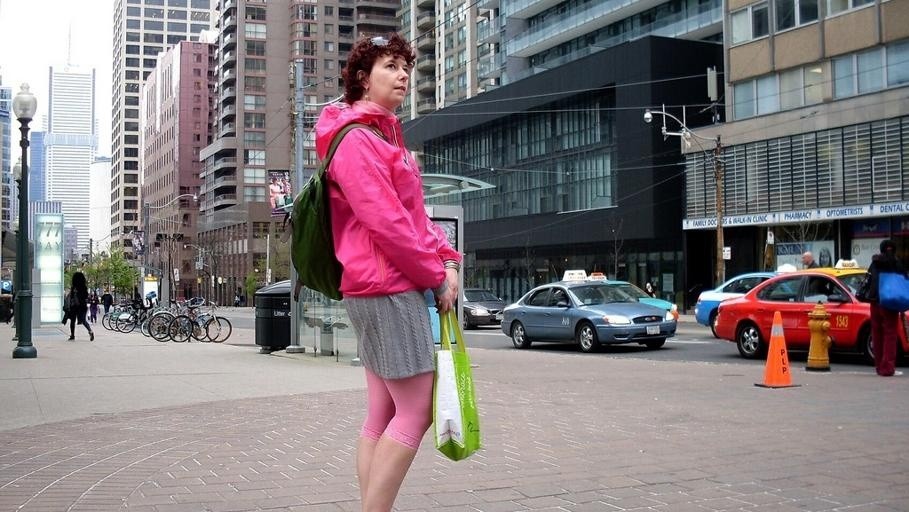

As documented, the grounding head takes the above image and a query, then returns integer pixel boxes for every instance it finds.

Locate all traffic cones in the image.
[755,311,802,388]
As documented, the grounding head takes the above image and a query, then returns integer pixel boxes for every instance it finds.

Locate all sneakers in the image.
[67,319,97,342]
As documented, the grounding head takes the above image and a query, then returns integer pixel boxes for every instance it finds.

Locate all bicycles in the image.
[102,292,232,343]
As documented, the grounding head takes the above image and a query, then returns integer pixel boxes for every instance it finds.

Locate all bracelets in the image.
[433,280,448,295]
[443,260,461,272]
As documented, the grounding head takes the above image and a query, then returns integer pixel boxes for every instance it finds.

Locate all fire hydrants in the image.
[805,301,832,371]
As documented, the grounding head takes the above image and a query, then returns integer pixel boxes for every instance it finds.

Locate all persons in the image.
[868,239,904,376]
[67,272,95,342]
[280,177,292,205]
[100,288,113,319]
[645,282,656,299]
[800,251,819,270]
[269,176,281,212]
[313,30,464,512]
[88,290,99,323]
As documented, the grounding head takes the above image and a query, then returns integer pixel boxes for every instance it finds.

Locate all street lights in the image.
[12,83,37,358]
[144,193,198,264]
[643,109,724,289]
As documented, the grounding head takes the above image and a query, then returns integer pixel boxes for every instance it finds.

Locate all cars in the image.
[463,270,678,352]
[695,259,909,367]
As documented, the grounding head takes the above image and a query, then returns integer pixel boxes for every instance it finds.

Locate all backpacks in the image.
[283,122,388,304]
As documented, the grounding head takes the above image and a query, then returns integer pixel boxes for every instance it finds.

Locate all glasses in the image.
[358,34,392,49]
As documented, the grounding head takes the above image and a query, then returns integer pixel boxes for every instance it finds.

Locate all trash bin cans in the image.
[254,279,292,354]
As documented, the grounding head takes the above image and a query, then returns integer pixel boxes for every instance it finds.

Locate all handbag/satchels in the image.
[428,297,483,462]
